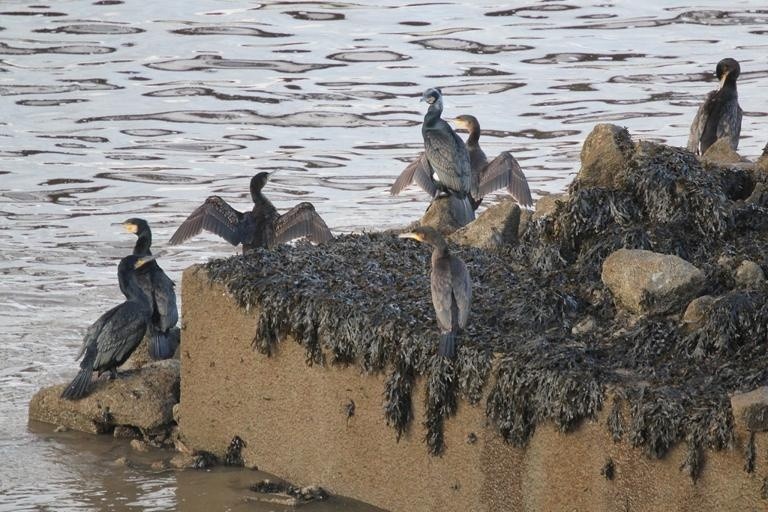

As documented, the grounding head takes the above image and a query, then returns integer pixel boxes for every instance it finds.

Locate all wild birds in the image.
[686,57,744,156]
[60,249,168,401]
[110,217,179,361]
[388,88,534,226]
[167,170,337,257]
[396,224,474,356]
